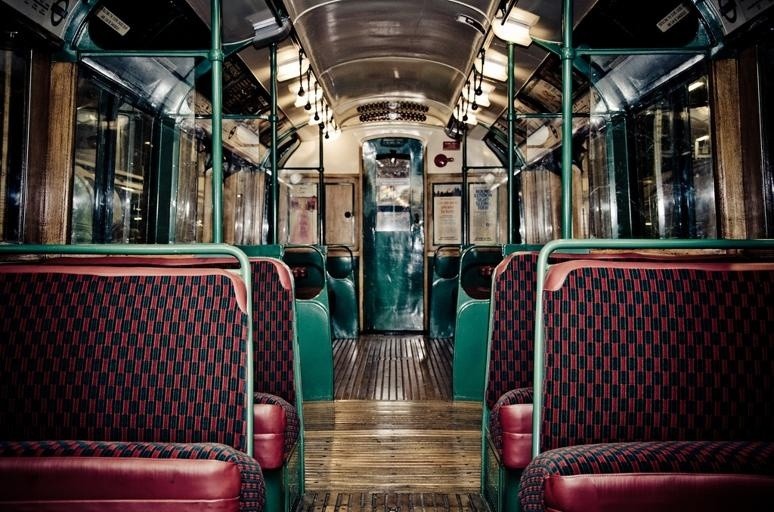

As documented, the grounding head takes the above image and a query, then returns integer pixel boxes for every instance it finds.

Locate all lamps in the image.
[461,84,491,107]
[491,2,538,47]
[468,74,496,94]
[474,48,508,81]
[453,109,478,126]
[277,44,341,142]
[457,96,483,116]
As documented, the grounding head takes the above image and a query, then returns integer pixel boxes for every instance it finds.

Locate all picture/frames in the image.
[432,182,464,246]
[287,182,318,247]
[468,182,499,245]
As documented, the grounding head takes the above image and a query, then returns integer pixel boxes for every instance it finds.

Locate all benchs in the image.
[229,244,336,401]
[429,242,503,338]
[0,265,267,511]
[453,244,543,402]
[480,251,768,510]
[0,241,304,508]
[283,241,359,338]
[518,259,770,510]
